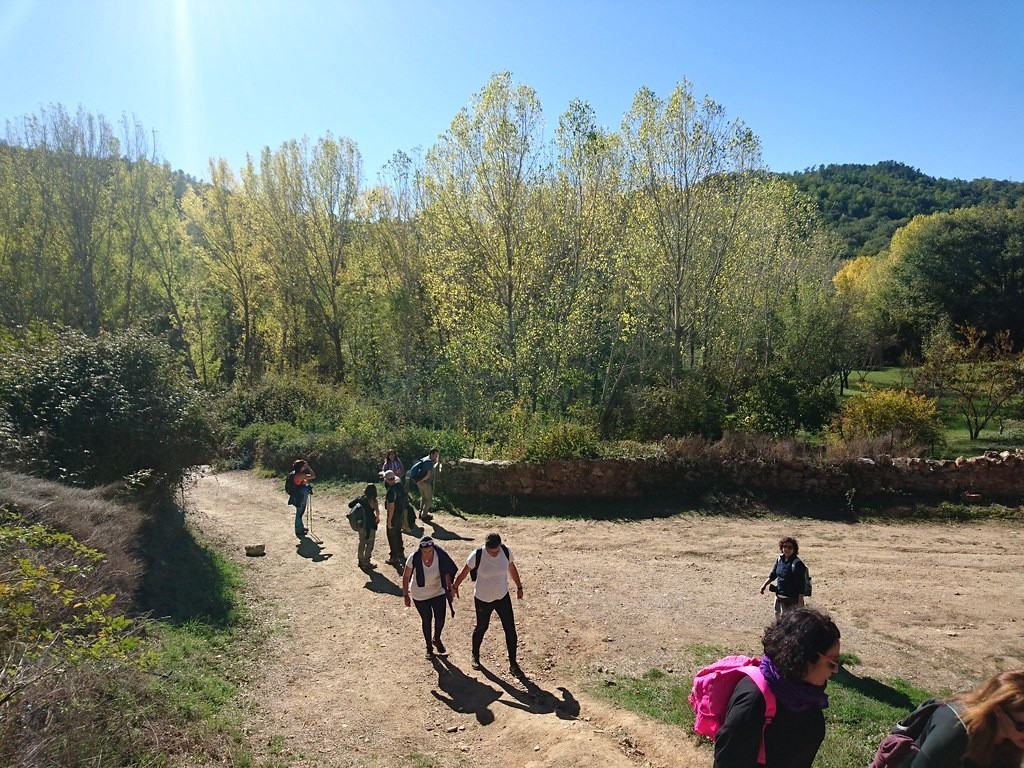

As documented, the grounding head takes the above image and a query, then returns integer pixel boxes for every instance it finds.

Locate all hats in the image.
[385,470,395,479]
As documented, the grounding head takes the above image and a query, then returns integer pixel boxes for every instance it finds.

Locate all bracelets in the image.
[517,586,522,590]
[404,594,408,597]
[453,584,458,590]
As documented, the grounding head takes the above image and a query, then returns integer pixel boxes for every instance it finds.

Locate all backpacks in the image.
[408,457,431,479]
[890,698,972,746]
[778,553,813,596]
[348,494,369,531]
[285,470,297,494]
[688,655,776,765]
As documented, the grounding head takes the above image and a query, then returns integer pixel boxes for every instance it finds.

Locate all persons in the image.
[348,482,380,569]
[293,460,315,535]
[385,470,407,564]
[760,537,806,617]
[403,536,452,658]
[869,669,1024,768]
[383,449,403,490]
[413,448,439,520]
[712,608,841,768]
[450,533,524,678]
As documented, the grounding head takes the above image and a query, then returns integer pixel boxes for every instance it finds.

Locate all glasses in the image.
[420,540,434,546]
[819,653,840,668]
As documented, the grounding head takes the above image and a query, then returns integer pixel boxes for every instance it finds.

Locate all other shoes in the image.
[418,510,433,520]
[303,528,309,532]
[508,664,523,678]
[426,644,434,659]
[433,637,446,653]
[296,531,307,535]
[471,657,481,670]
